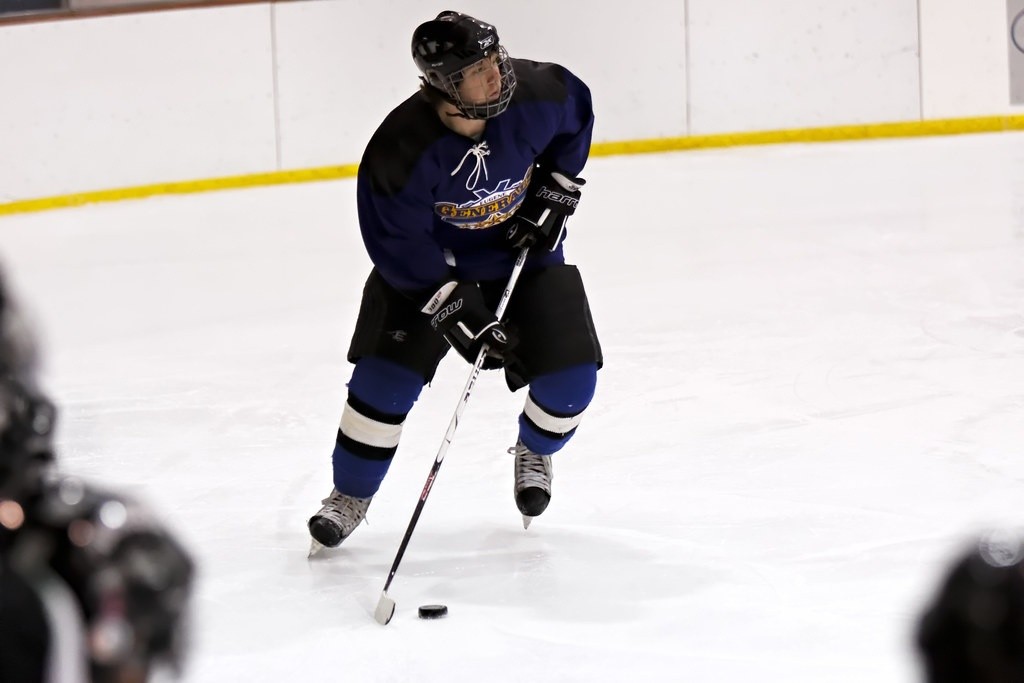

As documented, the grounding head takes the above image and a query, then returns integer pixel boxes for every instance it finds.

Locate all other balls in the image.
[417,604,448,618]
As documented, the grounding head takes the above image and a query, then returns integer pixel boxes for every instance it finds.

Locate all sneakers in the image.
[508,436,553,530]
[307,487,373,557]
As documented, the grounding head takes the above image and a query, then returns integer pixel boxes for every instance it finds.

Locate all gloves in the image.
[507,169,586,253]
[419,271,511,371]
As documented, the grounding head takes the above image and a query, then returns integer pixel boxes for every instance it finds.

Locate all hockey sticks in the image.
[371,246,538,627]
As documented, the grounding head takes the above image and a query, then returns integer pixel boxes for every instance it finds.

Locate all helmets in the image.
[411,9,517,121]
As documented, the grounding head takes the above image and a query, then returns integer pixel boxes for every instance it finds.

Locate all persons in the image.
[307,11,602,558]
